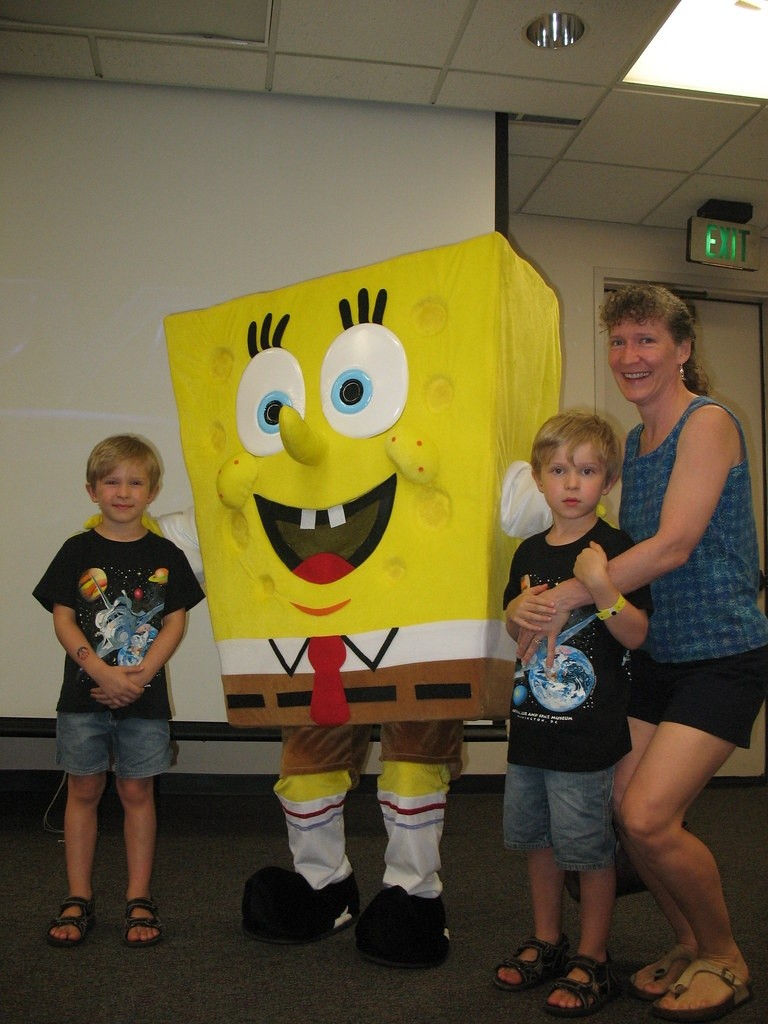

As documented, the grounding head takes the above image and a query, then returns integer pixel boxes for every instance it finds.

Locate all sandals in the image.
[122,896,162,947]
[543,953,618,1019]
[490,933,570,991]
[45,895,96,948]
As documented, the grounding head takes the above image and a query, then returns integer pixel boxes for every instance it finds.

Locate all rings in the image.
[533,638,542,645]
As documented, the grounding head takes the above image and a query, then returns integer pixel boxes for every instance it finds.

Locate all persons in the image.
[32,436,206,948]
[493,411,653,1017]
[515,284,768,1024]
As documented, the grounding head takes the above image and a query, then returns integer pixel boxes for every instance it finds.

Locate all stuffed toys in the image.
[86,232,607,968]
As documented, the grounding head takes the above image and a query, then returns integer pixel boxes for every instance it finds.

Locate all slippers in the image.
[625,943,695,1002]
[650,957,753,1023]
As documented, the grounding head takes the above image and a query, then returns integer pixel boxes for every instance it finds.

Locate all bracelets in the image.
[595,594,625,620]
